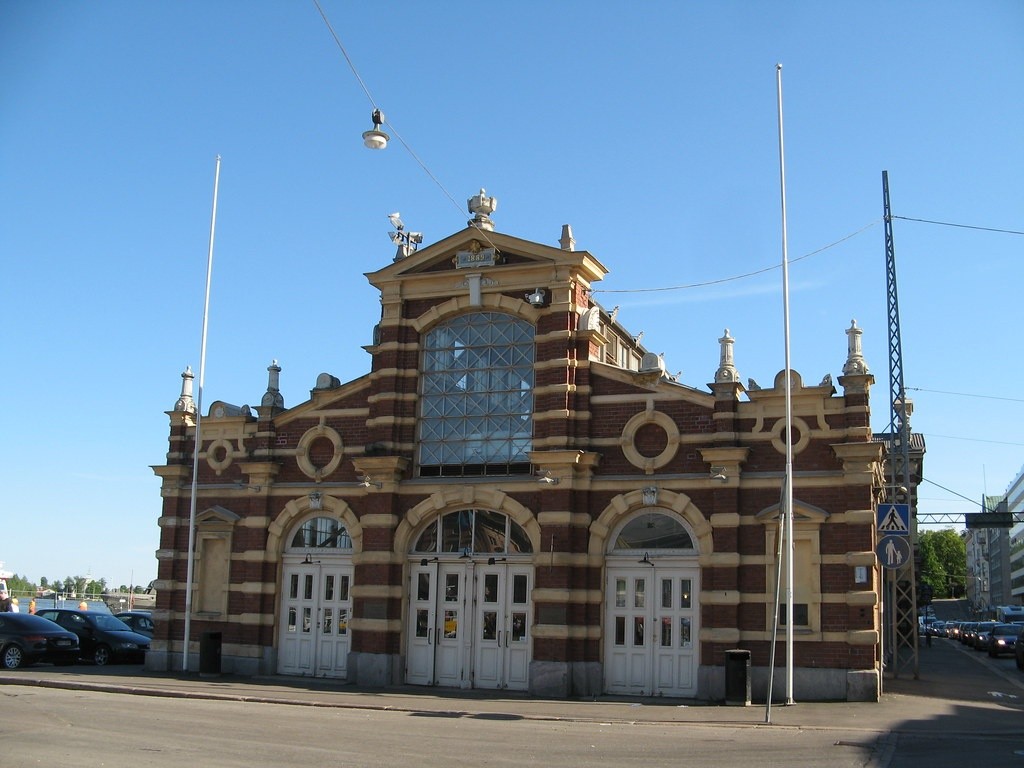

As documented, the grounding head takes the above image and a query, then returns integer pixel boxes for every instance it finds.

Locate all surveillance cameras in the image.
[529,293,543,308]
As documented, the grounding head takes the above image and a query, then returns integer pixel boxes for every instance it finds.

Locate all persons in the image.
[923,629,934,648]
[79,598,88,611]
[12,595,20,612]
[29,597,38,614]
[0,589,14,613]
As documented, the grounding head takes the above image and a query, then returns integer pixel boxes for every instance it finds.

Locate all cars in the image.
[34,609,151,666]
[114,612,155,640]
[918,605,1024,674]
[0,612,80,668]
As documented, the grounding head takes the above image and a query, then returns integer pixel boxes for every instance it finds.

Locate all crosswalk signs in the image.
[875,503,911,538]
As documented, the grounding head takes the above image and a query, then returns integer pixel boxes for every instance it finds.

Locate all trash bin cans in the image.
[199,631,223,678]
[724,649,752,706]
